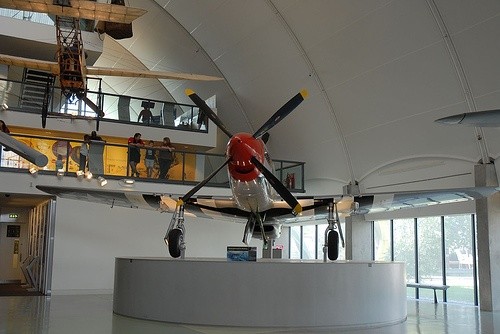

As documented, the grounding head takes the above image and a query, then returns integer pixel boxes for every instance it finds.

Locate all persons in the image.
[127,132,177,180]
[79,130,107,172]
[138,107,155,123]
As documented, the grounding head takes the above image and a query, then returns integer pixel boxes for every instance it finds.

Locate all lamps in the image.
[98,176,107,187]
[123,178,136,184]
[57,169,64,178]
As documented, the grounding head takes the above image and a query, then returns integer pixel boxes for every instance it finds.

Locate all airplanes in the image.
[0,0,148,132]
[35,88,500,260]
